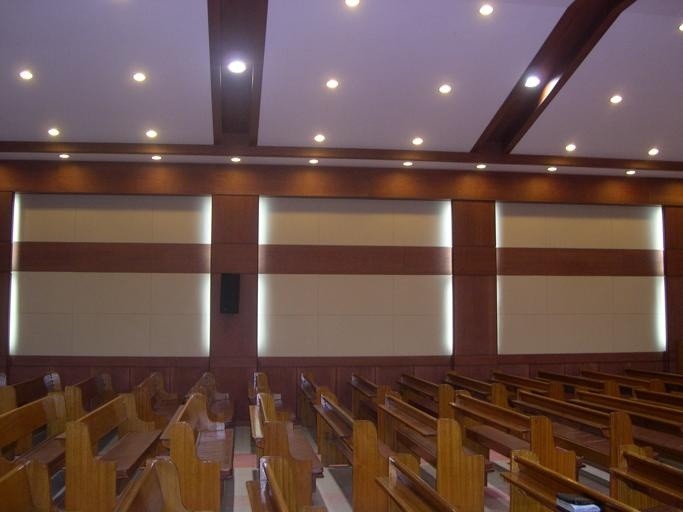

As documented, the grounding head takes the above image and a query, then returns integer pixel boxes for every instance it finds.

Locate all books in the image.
[554,491,601,512]
